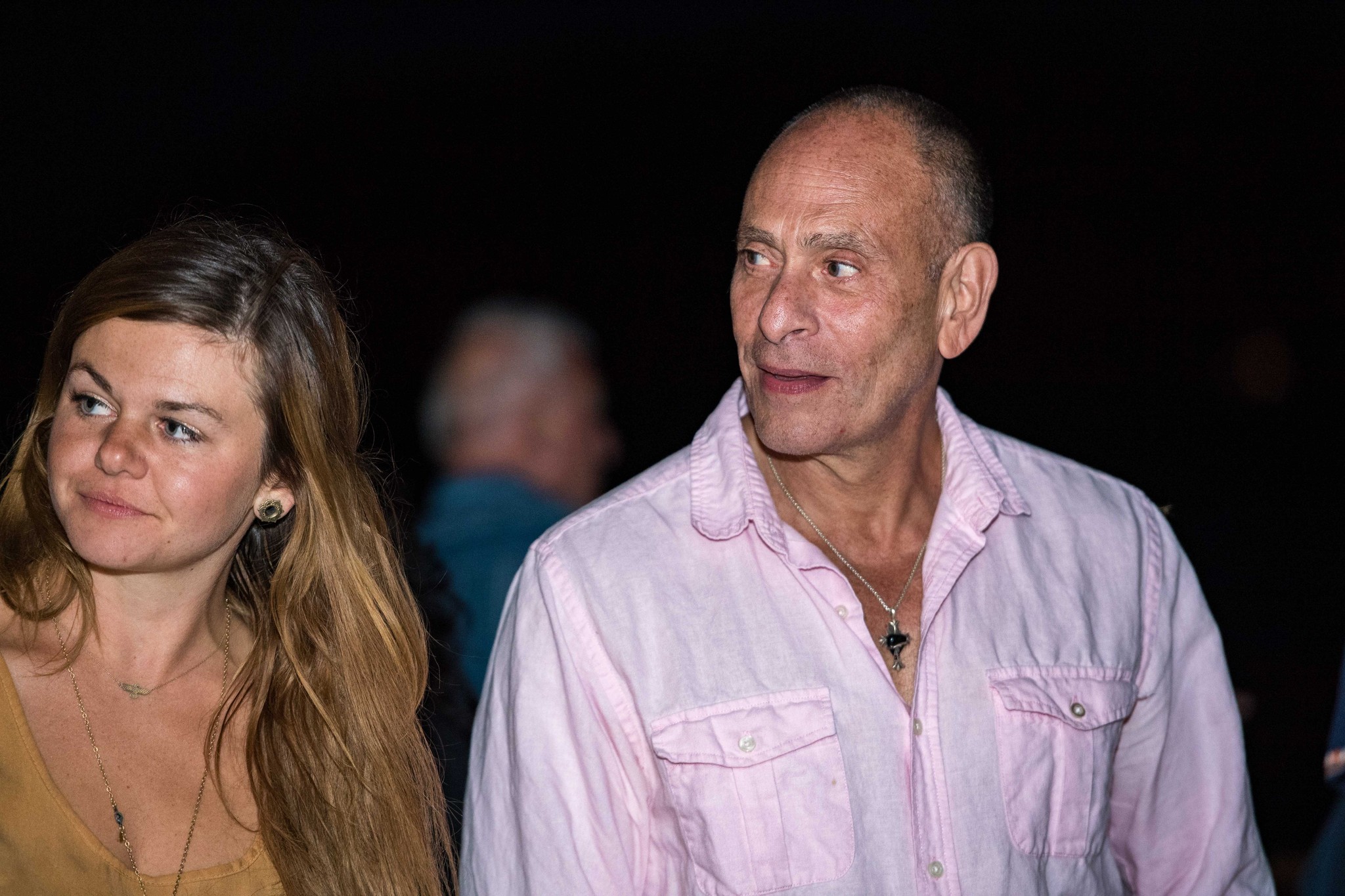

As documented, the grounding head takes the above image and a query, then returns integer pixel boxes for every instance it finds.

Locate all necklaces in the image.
[45,563,229,896]
[766,452,927,669]
[36,559,229,699]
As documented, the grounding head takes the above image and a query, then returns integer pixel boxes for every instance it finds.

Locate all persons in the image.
[0,222,456,896]
[463,91,1276,896]
[427,310,606,694]
